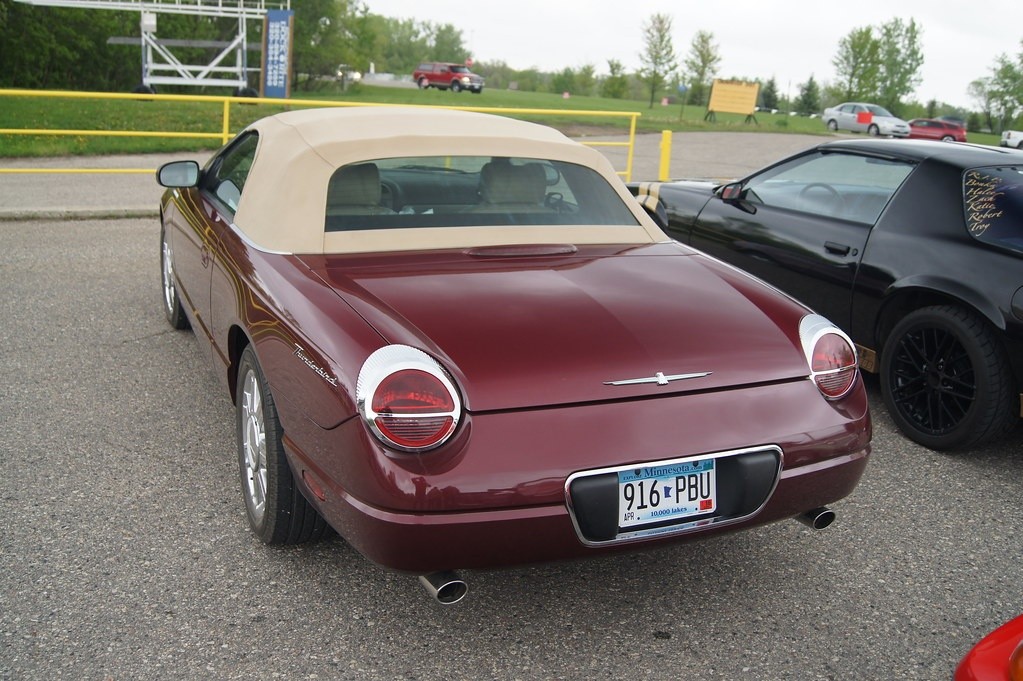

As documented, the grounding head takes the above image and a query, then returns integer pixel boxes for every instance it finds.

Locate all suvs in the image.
[411,61,485,94]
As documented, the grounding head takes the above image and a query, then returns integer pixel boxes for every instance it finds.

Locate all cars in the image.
[822,101,912,136]
[894,117,968,142]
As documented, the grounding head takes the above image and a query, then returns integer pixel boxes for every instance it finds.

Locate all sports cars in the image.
[616,138,1023,452]
[153,105,875,608]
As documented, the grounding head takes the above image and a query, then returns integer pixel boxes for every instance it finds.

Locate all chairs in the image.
[326,162,397,218]
[478,162,548,216]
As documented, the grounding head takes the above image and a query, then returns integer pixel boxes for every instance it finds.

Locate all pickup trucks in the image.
[1000,130,1023,148]
